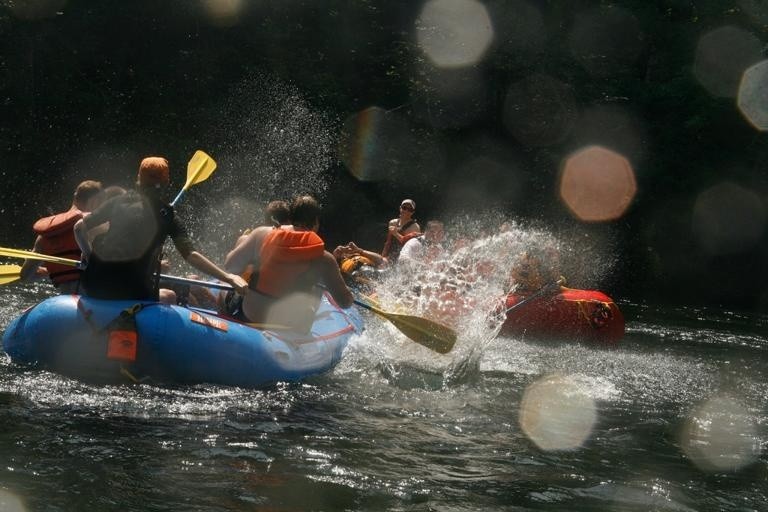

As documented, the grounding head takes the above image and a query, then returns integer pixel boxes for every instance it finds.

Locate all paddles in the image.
[0,264,51,285]
[0,247,234,290]
[316,284,458,354]
[172,150,217,207]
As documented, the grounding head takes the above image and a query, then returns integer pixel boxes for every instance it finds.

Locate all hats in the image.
[400,198,416,209]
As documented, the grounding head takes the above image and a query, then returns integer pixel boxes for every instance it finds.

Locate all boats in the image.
[381,282,624,346]
[2,279,365,390]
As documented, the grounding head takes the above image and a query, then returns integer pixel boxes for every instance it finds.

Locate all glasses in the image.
[402,205,413,212]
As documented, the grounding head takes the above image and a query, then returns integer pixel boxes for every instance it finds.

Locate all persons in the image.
[216,193,354,336]
[19,181,225,310]
[74,158,249,304]
[334,199,560,289]
